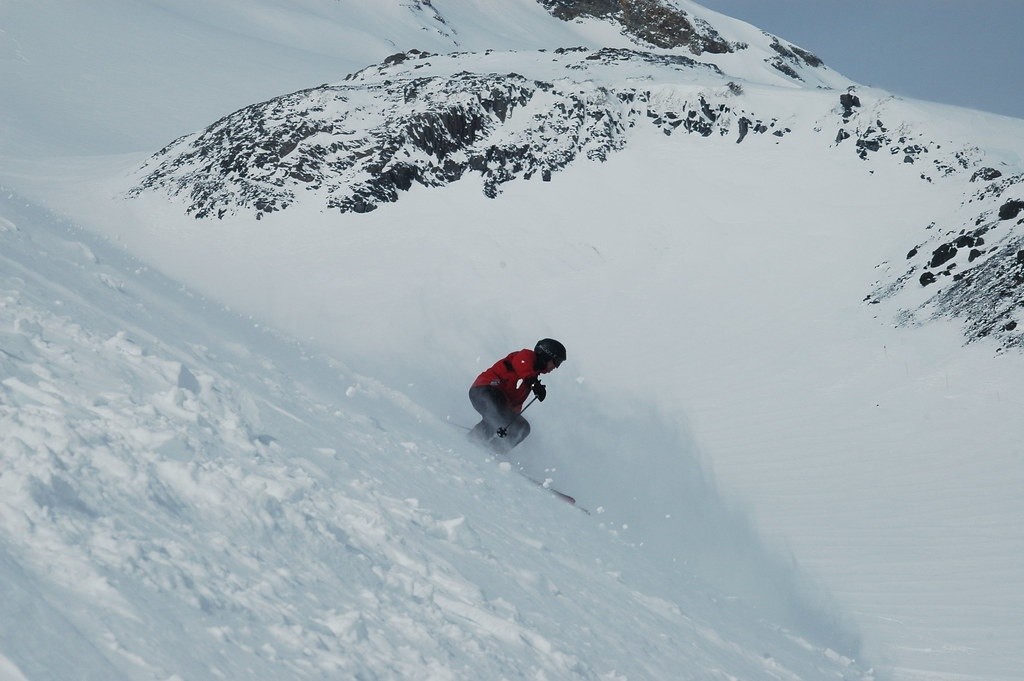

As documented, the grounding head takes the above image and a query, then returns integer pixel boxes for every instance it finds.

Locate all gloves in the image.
[532,384,546,401]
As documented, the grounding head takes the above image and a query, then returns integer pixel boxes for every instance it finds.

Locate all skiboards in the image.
[466,436,594,516]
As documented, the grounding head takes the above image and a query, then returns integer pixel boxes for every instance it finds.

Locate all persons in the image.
[468,339,566,456]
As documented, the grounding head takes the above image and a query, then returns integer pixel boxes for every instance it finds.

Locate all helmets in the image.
[535,338,566,368]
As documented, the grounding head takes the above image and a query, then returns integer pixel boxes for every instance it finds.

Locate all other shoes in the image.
[489,443,511,459]
[467,430,485,446]
